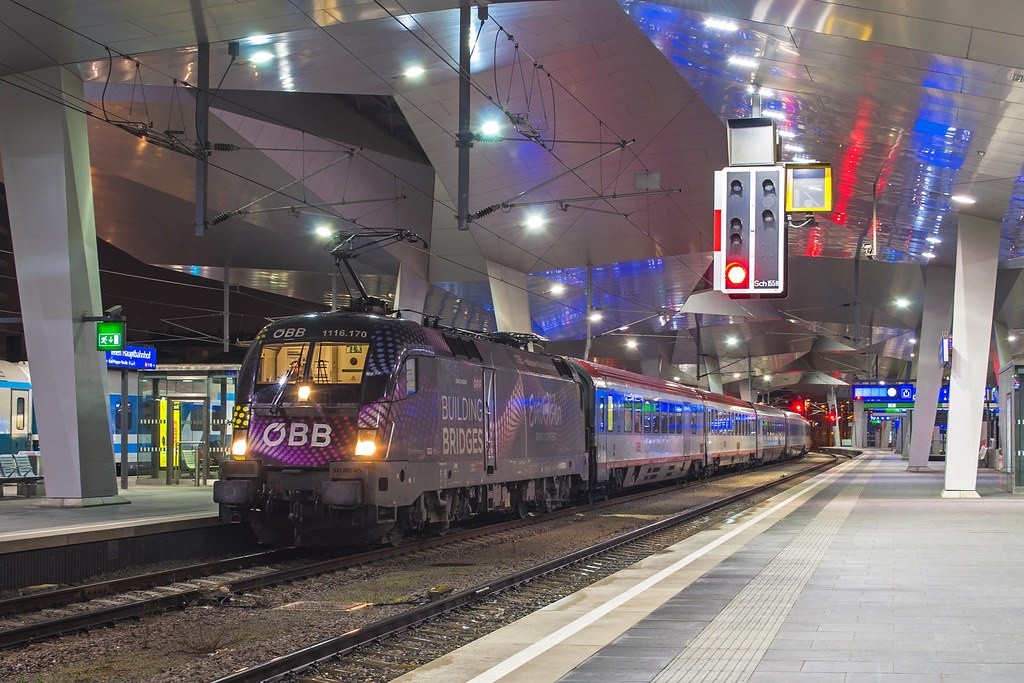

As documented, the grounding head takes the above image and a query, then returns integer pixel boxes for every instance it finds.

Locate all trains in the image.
[0,360,242,477]
[214,297,813,562]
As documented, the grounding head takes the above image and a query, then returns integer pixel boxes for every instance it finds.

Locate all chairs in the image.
[978,446,988,468]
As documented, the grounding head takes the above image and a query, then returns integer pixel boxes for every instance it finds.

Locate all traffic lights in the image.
[713,170,786,297]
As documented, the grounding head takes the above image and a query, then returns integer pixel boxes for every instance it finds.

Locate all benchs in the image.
[0,454,44,497]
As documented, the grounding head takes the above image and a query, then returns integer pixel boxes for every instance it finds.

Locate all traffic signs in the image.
[105,344,158,371]
